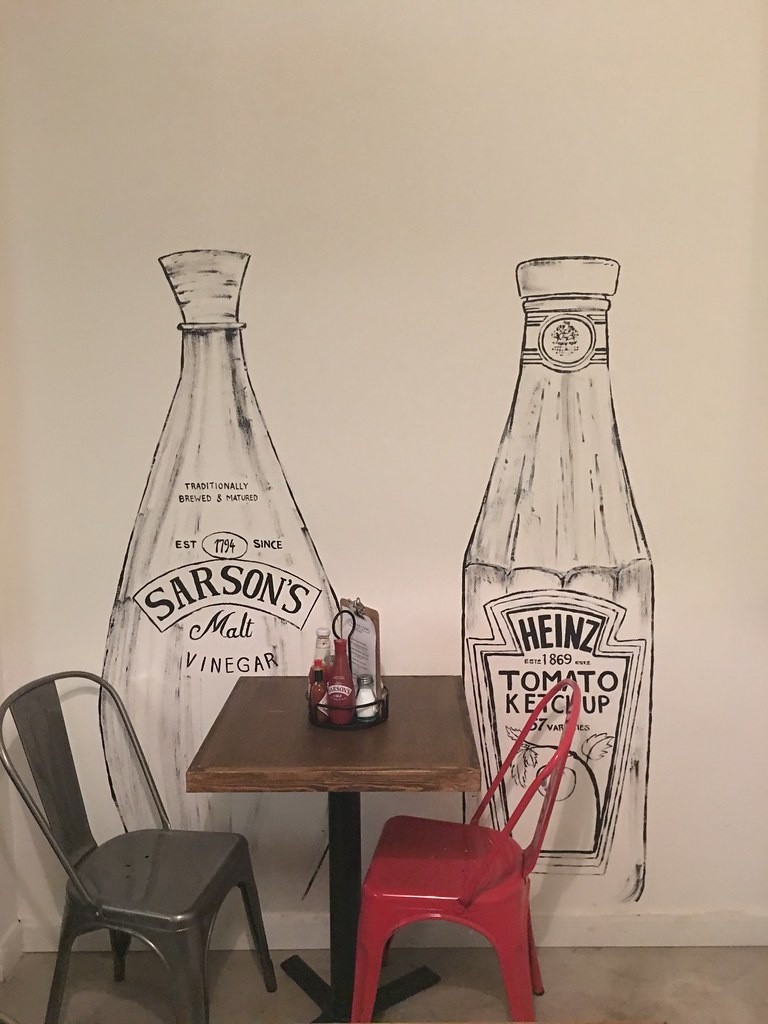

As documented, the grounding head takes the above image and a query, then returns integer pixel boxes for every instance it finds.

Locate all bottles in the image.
[308,627,389,725]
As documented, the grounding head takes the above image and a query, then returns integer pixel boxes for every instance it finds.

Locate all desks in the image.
[187,675,481,1023]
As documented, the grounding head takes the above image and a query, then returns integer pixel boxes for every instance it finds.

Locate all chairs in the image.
[353,680,582,1024]
[0,670,277,1024]
